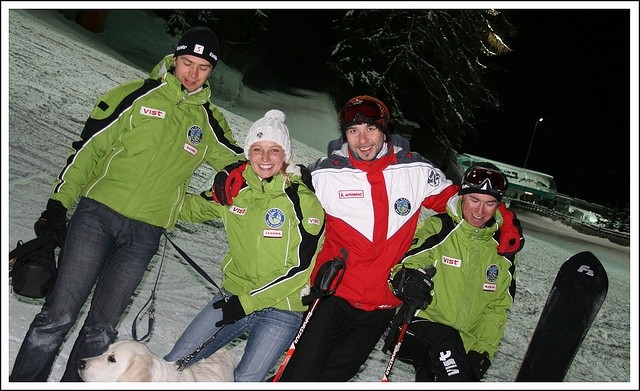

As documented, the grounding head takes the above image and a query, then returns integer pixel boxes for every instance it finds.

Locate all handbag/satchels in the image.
[9,238,56,298]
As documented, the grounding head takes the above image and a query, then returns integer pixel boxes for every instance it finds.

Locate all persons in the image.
[164,109,326,382]
[9,26,247,382]
[269,95,525,382]
[386,162,518,383]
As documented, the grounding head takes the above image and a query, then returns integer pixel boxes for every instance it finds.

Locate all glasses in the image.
[340,103,381,126]
[460,166,508,191]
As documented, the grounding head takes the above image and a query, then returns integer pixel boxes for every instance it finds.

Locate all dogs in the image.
[76,339,242,382]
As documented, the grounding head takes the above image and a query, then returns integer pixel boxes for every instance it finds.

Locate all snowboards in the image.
[511,250,609,381]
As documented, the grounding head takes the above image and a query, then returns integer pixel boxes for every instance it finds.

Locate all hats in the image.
[173,26,220,73]
[244,109,292,165]
[341,96,390,127]
[458,162,509,202]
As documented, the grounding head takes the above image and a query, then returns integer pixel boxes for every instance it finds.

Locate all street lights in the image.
[525,115,544,168]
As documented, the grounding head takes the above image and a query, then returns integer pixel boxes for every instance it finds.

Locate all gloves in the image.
[392,265,437,310]
[34,198,68,248]
[213,295,246,328]
[212,160,250,206]
[467,350,491,382]
